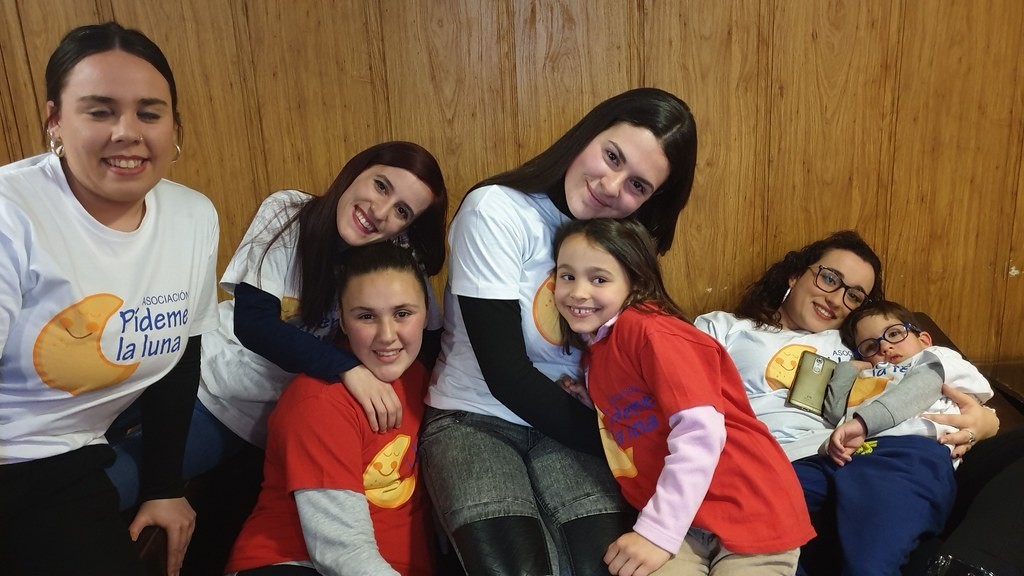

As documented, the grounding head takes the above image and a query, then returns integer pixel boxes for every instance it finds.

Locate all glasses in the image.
[807,264,873,311]
[854,323,922,360]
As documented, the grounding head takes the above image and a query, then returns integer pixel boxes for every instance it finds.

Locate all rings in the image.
[965,429,975,444]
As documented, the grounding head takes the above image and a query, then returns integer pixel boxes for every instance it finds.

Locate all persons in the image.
[107,141,447,576]
[694,229,1000,576]
[553,217,818,576]
[0,21,219,576]
[418,87,699,576]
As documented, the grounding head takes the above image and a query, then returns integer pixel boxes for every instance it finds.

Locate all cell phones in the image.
[787,350,836,416]
